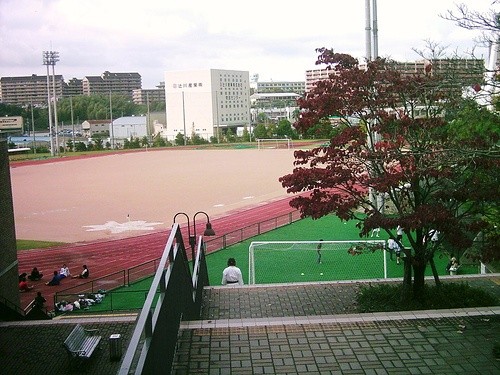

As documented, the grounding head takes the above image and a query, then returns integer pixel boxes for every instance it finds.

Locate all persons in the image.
[316,239,324,264]
[387,237,395,261]
[371,227,380,237]
[44,271,60,286]
[28,267,43,281]
[392,241,401,264]
[58,264,70,279]
[58,289,106,312]
[18,272,34,292]
[396,225,405,243]
[70,265,89,279]
[429,228,440,250]
[447,254,457,274]
[35,291,48,315]
[221,257,244,285]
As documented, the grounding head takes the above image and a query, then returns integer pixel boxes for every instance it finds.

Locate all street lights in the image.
[42,50,60,156]
[171,211,216,271]
